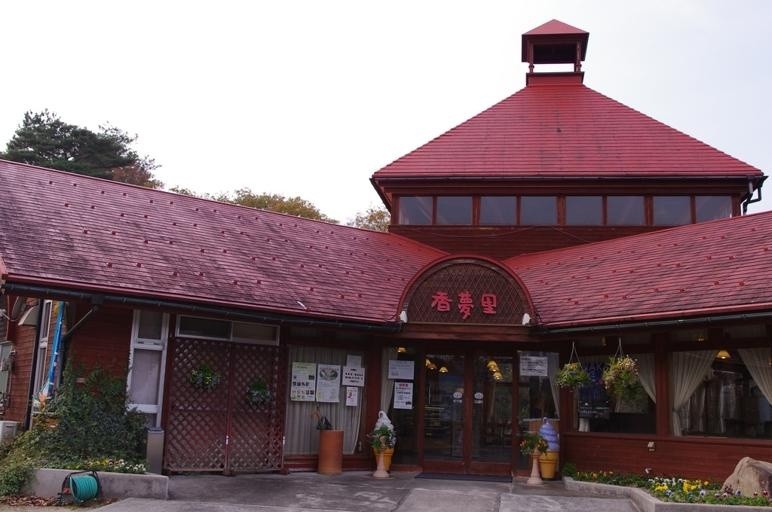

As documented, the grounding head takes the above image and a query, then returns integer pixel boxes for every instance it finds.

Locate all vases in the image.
[526,444,547,485]
[373,449,391,479]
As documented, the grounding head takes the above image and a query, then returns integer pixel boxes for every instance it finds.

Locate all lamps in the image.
[396,346,407,353]
[426,358,448,372]
[486,359,504,383]
[717,351,732,360]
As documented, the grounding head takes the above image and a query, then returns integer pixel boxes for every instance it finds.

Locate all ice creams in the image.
[538,417,560,479]
[373,410,396,471]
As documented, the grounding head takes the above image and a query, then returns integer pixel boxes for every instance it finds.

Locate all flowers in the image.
[190,363,221,388]
[519,429,550,457]
[597,355,643,400]
[365,423,396,453]
[246,380,274,407]
[552,361,592,390]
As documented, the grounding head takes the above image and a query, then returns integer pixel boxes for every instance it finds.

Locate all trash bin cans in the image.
[318,429,344,475]
